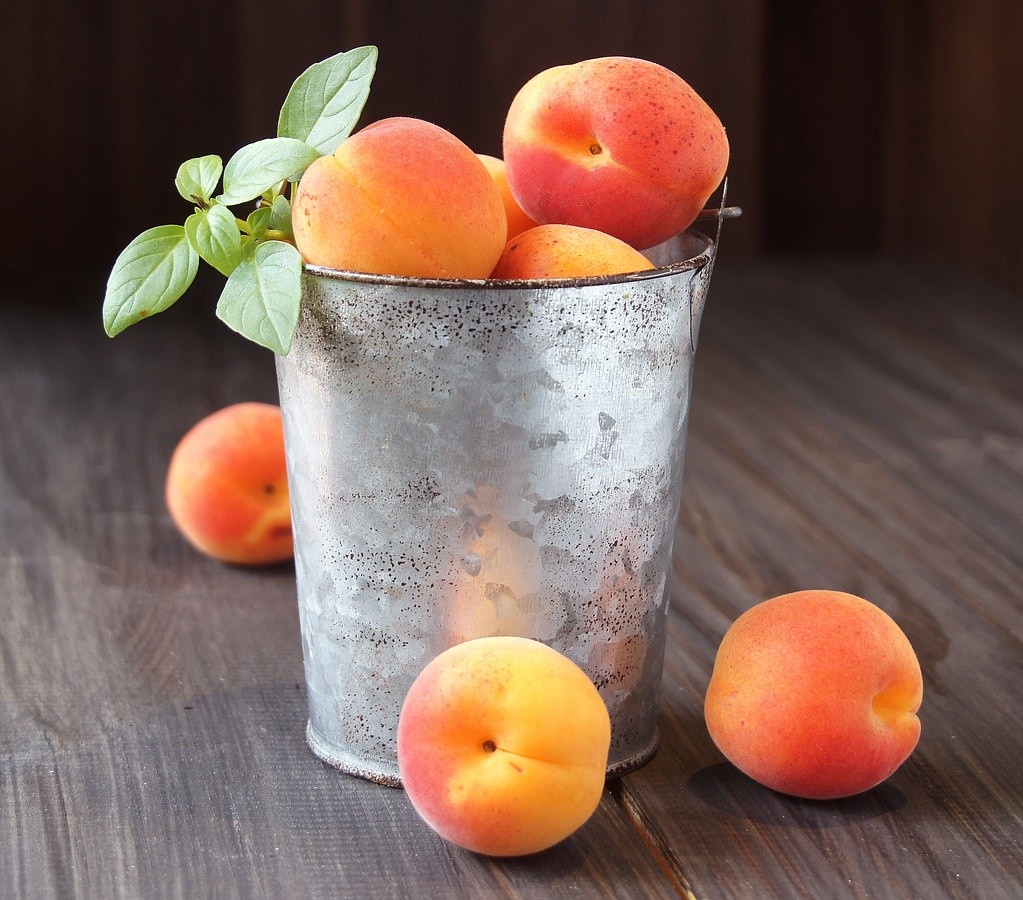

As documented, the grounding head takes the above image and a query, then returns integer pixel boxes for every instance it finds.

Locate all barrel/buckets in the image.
[271,176,743,788]
